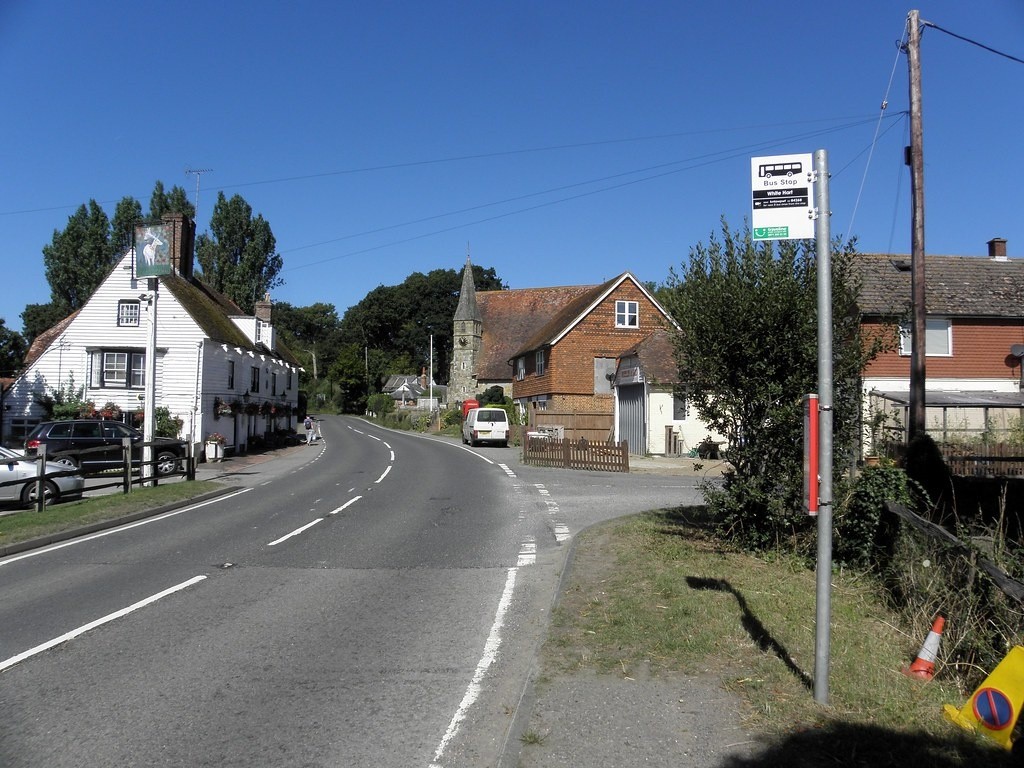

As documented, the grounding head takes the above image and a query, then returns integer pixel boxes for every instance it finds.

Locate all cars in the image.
[0,447,85,512]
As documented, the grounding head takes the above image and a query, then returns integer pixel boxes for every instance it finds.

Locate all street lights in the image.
[303,349,317,379]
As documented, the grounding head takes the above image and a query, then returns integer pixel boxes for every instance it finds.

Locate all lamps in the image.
[239,388,252,403]
[273,389,287,402]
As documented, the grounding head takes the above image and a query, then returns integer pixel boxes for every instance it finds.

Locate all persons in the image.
[304,415,317,446]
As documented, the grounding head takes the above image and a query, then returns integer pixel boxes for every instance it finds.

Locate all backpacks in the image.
[304,419,311,429]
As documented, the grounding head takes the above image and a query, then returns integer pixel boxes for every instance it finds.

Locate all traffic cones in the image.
[944,645,1024,757]
[901,615,945,681]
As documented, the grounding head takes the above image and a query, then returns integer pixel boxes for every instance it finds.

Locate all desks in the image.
[704,441,727,460]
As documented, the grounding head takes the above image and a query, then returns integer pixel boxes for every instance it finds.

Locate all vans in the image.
[460,407,510,448]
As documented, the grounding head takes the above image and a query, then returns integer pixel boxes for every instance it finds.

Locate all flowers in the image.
[270,406,277,414]
[218,400,232,415]
[208,432,226,444]
[132,405,145,422]
[79,399,97,418]
[98,402,121,419]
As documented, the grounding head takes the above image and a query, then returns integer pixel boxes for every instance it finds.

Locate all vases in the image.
[204,441,225,463]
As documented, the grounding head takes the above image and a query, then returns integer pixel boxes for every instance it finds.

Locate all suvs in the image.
[24,418,190,478]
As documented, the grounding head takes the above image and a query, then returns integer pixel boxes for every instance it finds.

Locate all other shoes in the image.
[307,442,310,446]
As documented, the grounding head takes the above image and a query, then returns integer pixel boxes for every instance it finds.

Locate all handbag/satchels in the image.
[312,433,316,441]
